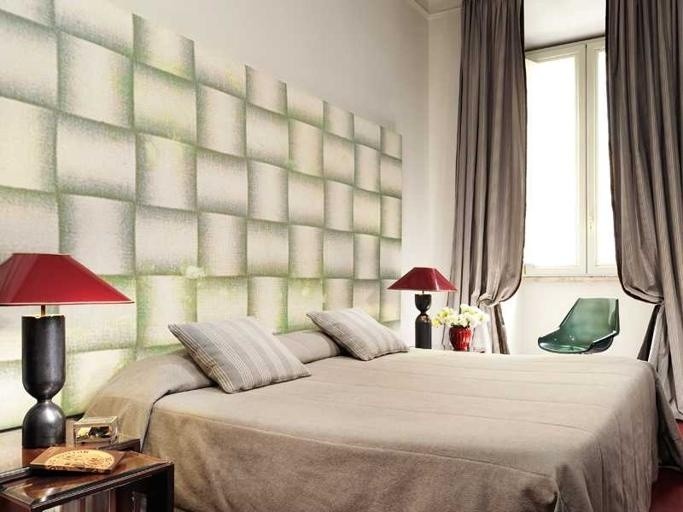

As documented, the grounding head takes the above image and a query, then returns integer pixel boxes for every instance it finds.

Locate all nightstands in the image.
[0,417,141,512]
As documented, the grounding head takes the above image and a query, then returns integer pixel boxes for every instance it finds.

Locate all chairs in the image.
[537,297,620,355]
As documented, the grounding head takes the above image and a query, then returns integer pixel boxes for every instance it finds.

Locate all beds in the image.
[80,329,683,512]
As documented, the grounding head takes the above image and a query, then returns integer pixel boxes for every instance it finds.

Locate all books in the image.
[28,446,126,474]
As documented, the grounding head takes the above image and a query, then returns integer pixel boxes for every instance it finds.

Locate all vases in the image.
[448,325,472,352]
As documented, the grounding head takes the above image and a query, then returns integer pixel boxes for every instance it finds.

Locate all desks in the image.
[0,449,175,512]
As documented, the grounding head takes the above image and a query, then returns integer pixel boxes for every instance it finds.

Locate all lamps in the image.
[0,252,136,450]
[387,266,459,349]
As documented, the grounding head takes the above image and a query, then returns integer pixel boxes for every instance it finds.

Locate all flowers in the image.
[431,303,492,328]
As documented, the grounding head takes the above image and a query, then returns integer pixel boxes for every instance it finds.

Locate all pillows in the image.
[167,315,312,394]
[99,329,342,406]
[305,307,411,362]
